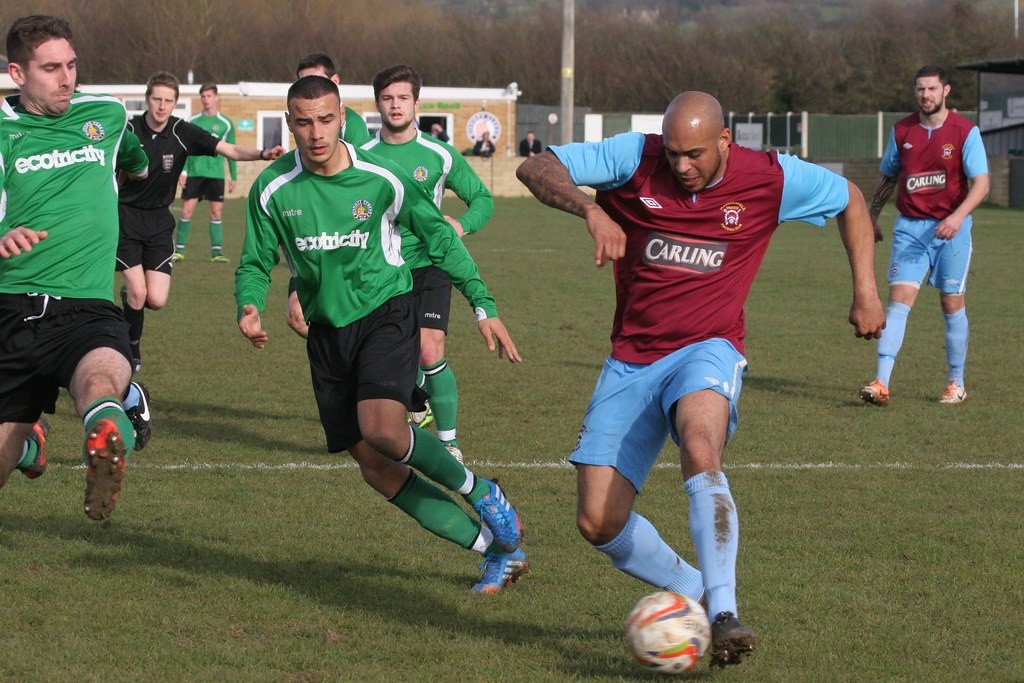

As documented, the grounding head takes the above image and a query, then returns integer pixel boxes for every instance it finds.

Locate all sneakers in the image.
[469,547,531,595]
[858,379,890,407]
[18,415,51,480]
[209,255,230,264]
[83,419,126,521]
[125,381,153,452]
[445,446,463,466]
[170,252,186,264]
[697,588,756,668]
[408,407,434,429]
[939,379,967,404]
[473,479,525,552]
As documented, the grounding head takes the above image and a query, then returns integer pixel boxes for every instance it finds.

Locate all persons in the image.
[116,15,497,451]
[860,65,990,406]
[515,90,887,666]
[233,75,532,596]
[0,15,151,522]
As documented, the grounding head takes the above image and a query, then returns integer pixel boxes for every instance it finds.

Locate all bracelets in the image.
[260,149,270,161]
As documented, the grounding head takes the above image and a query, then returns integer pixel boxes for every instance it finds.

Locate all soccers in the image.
[623,590,712,674]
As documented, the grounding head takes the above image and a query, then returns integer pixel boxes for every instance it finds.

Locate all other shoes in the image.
[131,352,142,375]
[120,285,127,307]
